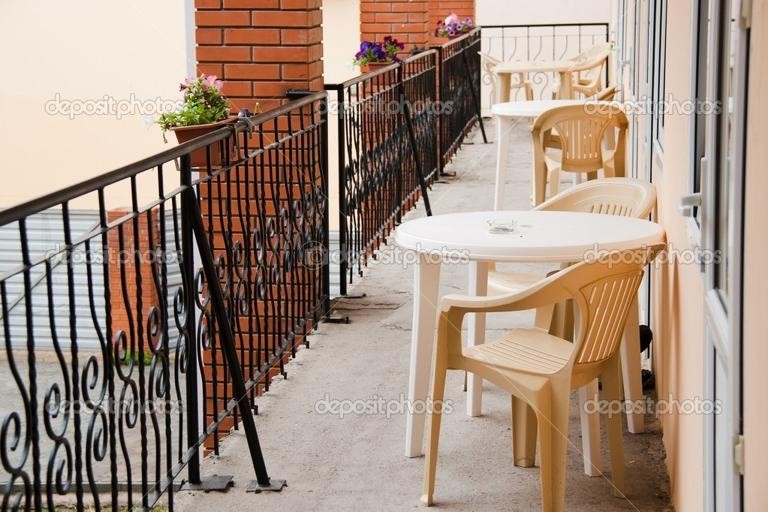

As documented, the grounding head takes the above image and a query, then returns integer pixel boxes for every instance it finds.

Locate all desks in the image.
[491,100,623,210]
[391,210,666,506]
[496,61,576,102]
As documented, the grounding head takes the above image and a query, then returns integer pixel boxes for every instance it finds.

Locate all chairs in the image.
[466,176,662,419]
[477,52,535,102]
[531,102,631,208]
[552,43,614,100]
[421,238,670,512]
[584,87,615,102]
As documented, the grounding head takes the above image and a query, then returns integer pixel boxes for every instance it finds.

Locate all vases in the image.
[367,58,398,87]
[170,116,238,167]
[447,33,474,52]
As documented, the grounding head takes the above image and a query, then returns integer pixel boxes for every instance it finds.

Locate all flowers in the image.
[352,35,405,69]
[432,13,475,38]
[155,74,230,143]
